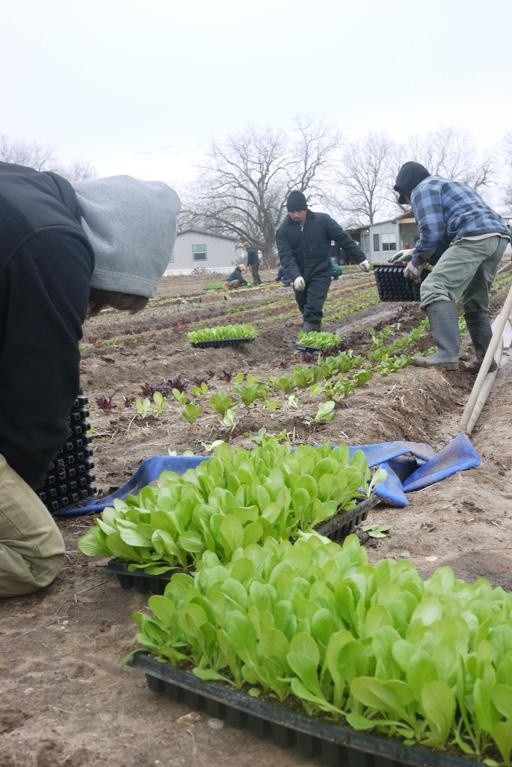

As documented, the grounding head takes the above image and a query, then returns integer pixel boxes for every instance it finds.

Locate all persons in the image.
[275,263,291,287]
[275,190,371,353]
[0,161,181,599]
[225,264,247,289]
[330,260,342,280]
[388,160,512,373]
[242,241,261,286]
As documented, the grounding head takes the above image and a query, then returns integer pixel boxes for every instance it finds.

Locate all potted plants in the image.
[295,330,344,354]
[202,281,223,290]
[121,534,512,767]
[187,323,257,348]
[76,439,381,595]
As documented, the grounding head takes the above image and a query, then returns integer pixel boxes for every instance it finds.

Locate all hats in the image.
[240,264,246,270]
[241,242,251,248]
[393,185,406,204]
[287,191,307,212]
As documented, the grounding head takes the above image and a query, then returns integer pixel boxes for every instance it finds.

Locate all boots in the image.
[411,300,459,371]
[459,310,497,373]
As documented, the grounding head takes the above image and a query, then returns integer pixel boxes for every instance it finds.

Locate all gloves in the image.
[294,277,305,292]
[403,261,426,280]
[388,248,416,263]
[360,259,370,272]
